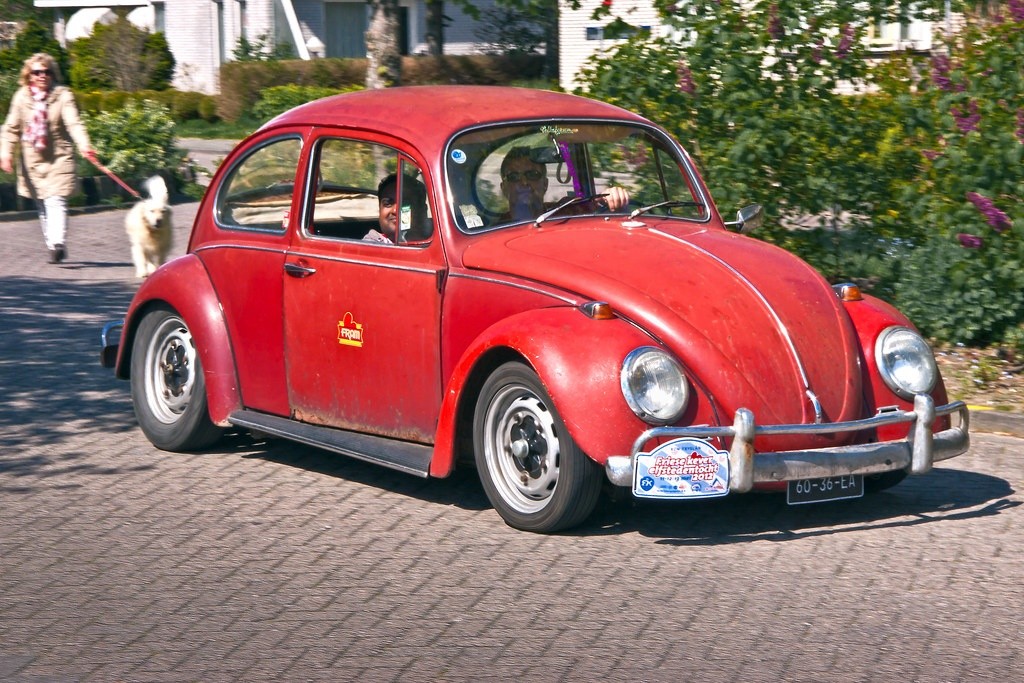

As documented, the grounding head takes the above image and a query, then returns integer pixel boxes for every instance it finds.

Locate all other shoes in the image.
[49,250,59,264]
[54,244,68,259]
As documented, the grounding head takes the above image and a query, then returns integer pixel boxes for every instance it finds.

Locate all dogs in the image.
[126,174,173,278]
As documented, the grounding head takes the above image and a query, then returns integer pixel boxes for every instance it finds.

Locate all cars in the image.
[99,84,971,532]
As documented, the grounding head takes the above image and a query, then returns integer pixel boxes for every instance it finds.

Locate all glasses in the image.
[30,69,53,76]
[502,170,544,182]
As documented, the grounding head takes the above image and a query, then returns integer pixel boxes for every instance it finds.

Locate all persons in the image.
[0,53,93,264]
[496,146,630,226]
[361,172,427,248]
